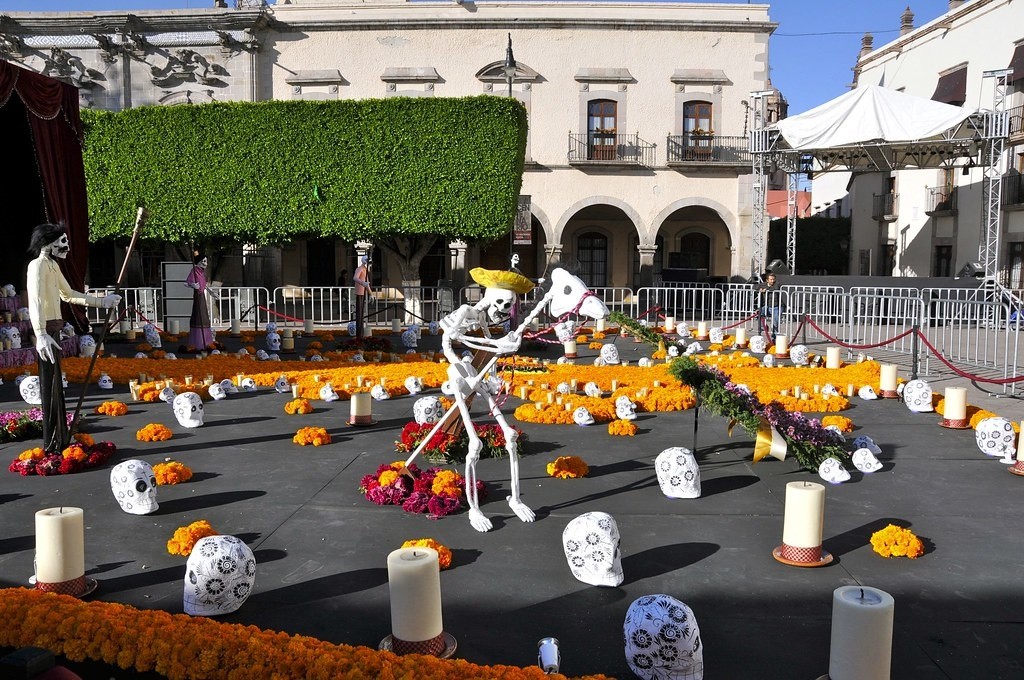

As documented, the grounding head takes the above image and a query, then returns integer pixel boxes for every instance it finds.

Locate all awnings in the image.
[998,42,1024,86]
[930,67,966,105]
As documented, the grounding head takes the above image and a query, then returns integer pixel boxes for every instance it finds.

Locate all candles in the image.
[284,338,294,352]
[1017,420,1024,460]
[736,328,746,345]
[776,335,787,354]
[35,504,85,597]
[944,385,967,419]
[350,392,371,424]
[304,320,314,334]
[697,321,707,336]
[387,547,445,657]
[829,585,894,679]
[564,341,577,357]
[364,328,372,336]
[232,319,240,334]
[827,345,842,369]
[665,316,674,331]
[880,362,897,391]
[392,319,401,333]
[283,329,293,338]
[530,317,539,332]
[171,319,179,335]
[782,480,826,562]
[596,319,606,332]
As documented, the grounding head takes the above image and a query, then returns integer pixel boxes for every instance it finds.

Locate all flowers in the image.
[596,127,617,134]
[871,522,923,560]
[168,518,219,556]
[399,537,451,567]
[0,327,1020,476]
[151,462,193,486]
[358,459,484,519]
[689,127,716,136]
[0,588,618,680]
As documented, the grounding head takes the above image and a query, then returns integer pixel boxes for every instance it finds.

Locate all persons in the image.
[338,269,350,301]
[757,273,781,345]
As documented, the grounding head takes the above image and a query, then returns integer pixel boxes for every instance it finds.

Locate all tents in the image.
[746,85,1007,324]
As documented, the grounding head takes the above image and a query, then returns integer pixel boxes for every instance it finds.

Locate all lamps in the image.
[806,169,812,180]
[537,637,561,674]
[769,154,778,174]
[962,164,969,175]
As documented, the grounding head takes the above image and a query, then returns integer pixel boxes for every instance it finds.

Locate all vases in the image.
[594,132,617,139]
[690,135,714,140]
